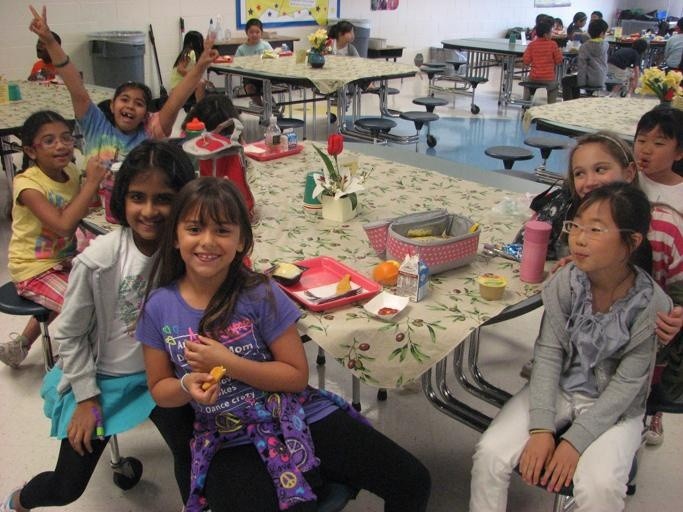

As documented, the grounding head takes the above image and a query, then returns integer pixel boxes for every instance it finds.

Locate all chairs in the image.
[414,70,442,113]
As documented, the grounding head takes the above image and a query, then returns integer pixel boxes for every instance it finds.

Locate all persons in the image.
[519,107,683,444]
[306,20,382,91]
[542,16,554,26]
[0,140,196,512]
[588,11,613,35]
[567,12,588,41]
[171,31,216,111]
[553,18,567,33]
[136,177,431,512]
[1,5,220,369]
[181,94,246,145]
[235,18,276,106]
[531,14,548,40]
[561,19,609,101]
[469,180,674,512]
[664,16,683,76]
[551,130,683,413]
[8,110,112,322]
[27,31,61,81]
[522,22,563,119]
[607,38,648,99]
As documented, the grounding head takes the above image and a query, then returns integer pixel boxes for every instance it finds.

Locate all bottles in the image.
[265,116,281,155]
[185,118,206,171]
[103,162,123,224]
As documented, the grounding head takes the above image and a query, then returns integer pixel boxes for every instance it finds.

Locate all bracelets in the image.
[180,373,190,393]
[529,429,553,434]
[52,55,69,67]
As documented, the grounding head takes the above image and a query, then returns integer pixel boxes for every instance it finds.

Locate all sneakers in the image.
[2,331,30,371]
[641,412,667,449]
[1,487,21,512]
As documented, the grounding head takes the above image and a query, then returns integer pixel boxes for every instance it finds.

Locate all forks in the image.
[318,285,364,305]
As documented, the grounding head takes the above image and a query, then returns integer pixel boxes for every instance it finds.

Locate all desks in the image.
[442,37,555,100]
[211,50,419,133]
[2,75,124,202]
[528,93,678,174]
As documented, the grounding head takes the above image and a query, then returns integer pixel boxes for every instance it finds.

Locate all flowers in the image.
[306,29,328,47]
[635,62,682,99]
[311,133,356,210]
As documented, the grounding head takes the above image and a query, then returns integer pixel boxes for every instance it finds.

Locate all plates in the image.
[274,256,382,310]
[241,142,303,161]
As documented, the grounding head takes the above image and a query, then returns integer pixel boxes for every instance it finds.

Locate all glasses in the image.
[30,130,77,151]
[562,221,636,241]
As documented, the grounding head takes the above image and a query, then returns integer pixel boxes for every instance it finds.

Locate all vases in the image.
[660,100,682,107]
[306,48,328,68]
[321,191,358,222]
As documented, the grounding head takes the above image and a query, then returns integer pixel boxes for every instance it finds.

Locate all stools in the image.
[446,59,461,93]
[424,64,446,68]
[511,458,637,496]
[483,143,536,176]
[353,117,395,145]
[0,278,58,376]
[282,484,362,511]
[399,112,439,151]
[102,429,148,489]
[460,77,490,116]
[524,139,572,172]
[411,94,448,112]
[275,117,307,135]
